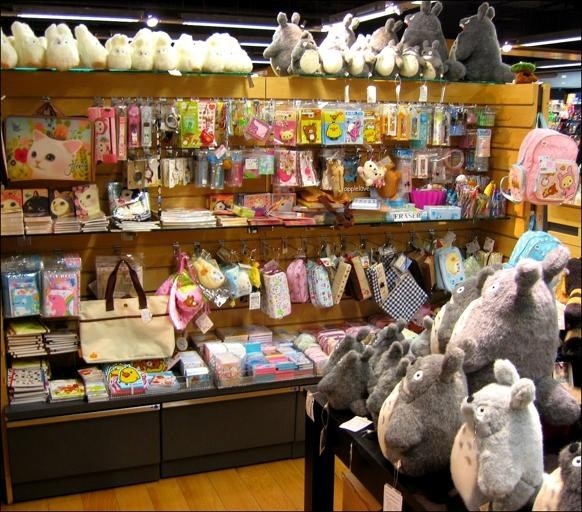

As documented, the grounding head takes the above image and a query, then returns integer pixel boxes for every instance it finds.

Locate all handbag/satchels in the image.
[78,260,175,364]
[366,255,428,324]
[0,101,96,189]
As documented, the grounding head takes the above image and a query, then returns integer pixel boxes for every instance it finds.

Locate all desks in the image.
[301,387,464,512]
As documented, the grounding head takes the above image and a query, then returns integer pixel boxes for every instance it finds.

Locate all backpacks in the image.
[507,215,563,292]
[500,112,580,207]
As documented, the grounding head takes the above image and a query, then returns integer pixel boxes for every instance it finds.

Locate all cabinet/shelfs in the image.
[1,68,550,507]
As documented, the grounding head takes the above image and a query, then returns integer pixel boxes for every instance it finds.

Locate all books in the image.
[6,324,80,358]
[105,362,180,399]
[1,188,25,235]
[160,206,217,230]
[210,193,316,228]
[74,183,110,232]
[23,189,53,235]
[51,190,81,234]
[181,321,309,386]
[5,360,109,405]
[109,183,161,232]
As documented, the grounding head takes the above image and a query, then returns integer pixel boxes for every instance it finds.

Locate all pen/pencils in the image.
[458,175,508,219]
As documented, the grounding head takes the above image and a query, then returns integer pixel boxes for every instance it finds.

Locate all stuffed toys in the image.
[553,257,581,386]
[445,250,581,424]
[399,1,466,82]
[288,30,448,80]
[263,12,302,76]
[368,19,403,56]
[320,12,359,51]
[1,0,515,84]
[0,20,253,75]
[430,262,503,354]
[316,316,581,510]
[309,248,581,512]
[450,3,515,84]
